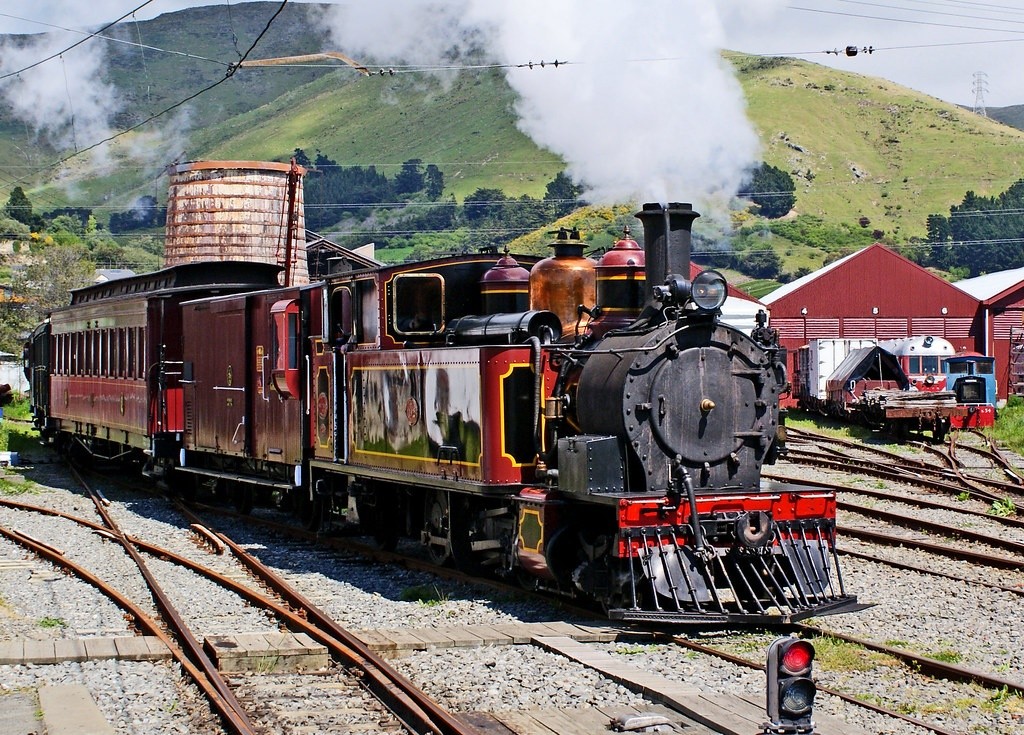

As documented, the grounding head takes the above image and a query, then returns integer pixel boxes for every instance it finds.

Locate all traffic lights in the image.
[766,638,815,732]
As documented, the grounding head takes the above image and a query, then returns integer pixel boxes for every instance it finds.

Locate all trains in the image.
[21,201,873,624]
